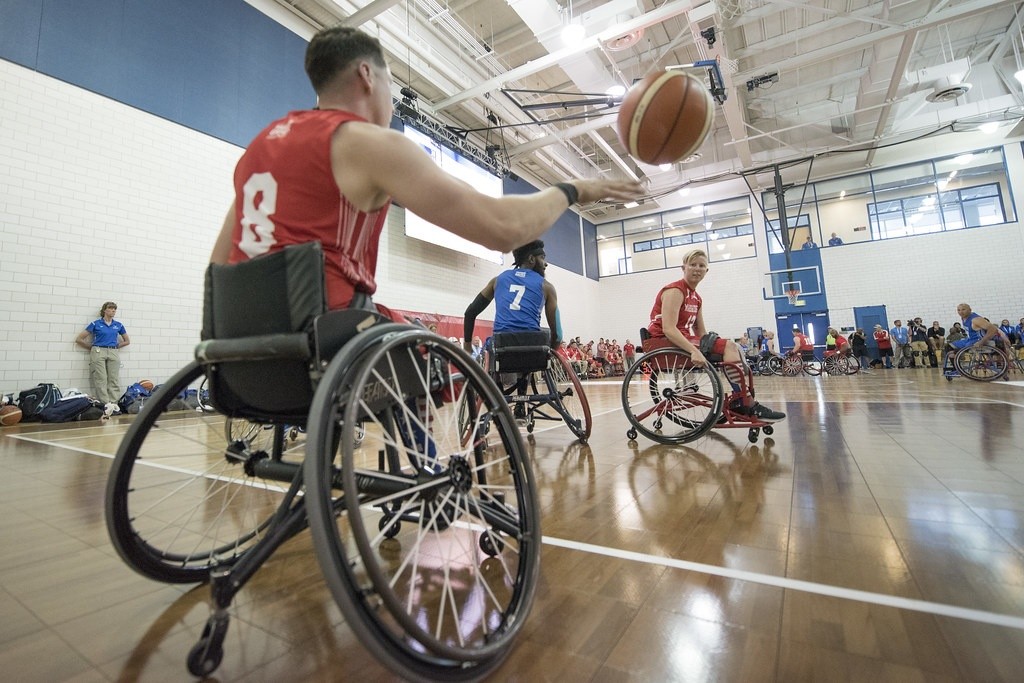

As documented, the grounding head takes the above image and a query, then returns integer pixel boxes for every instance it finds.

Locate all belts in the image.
[92,345,118,349]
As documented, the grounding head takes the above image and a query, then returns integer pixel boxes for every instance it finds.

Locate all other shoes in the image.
[111,411,122,415]
[945,371,961,376]
[514,403,526,418]
[867,365,874,369]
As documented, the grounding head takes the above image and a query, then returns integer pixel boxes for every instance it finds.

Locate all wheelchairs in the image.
[103,239,543,677]
[943,334,1011,382]
[456,330,593,451]
[621,327,775,445]
[783,345,822,377]
[757,350,787,377]
[823,347,860,376]
[744,355,761,376]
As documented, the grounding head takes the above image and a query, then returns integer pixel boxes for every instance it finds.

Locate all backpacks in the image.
[17,382,209,422]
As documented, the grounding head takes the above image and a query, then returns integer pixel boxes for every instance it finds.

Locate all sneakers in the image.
[740,403,785,422]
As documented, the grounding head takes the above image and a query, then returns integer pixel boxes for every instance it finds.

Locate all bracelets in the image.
[836,351,841,354]
[555,183,579,206]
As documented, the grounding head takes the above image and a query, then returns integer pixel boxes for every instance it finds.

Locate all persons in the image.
[944,303,1015,377]
[75,302,130,415]
[739,330,785,373]
[829,233,843,245]
[559,337,637,379]
[946,322,969,341]
[648,250,785,422]
[848,328,870,370]
[472,336,483,360]
[891,318,945,369]
[998,318,1024,359]
[802,237,817,249]
[785,329,814,359]
[430,324,437,332]
[464,239,565,423]
[823,327,848,361]
[873,325,896,369]
[208,26,648,393]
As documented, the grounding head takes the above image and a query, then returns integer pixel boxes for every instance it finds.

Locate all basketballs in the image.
[139,380,153,391]
[615,68,715,166]
[0,404,22,426]
